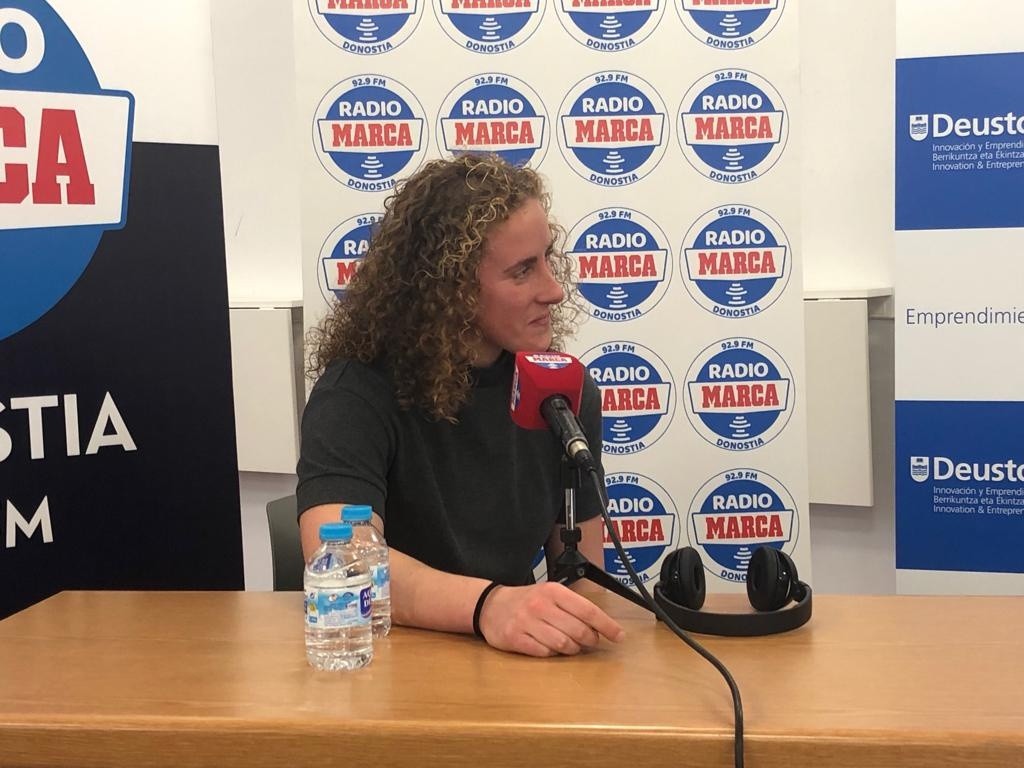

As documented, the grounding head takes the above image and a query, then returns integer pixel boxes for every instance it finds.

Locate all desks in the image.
[0,589,1024,768]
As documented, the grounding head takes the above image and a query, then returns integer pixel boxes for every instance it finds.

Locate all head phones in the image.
[655,547,814,636]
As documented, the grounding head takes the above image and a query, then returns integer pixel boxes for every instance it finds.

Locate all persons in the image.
[298,150,626,657]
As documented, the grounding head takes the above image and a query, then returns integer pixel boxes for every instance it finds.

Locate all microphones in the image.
[508,350,600,473]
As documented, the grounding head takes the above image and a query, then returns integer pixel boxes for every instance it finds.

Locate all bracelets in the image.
[473,577,509,640]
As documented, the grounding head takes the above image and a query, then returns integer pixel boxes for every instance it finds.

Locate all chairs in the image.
[264,493,307,592]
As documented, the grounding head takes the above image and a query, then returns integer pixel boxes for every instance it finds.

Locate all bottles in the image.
[304,525,375,671]
[337,505,392,641]
